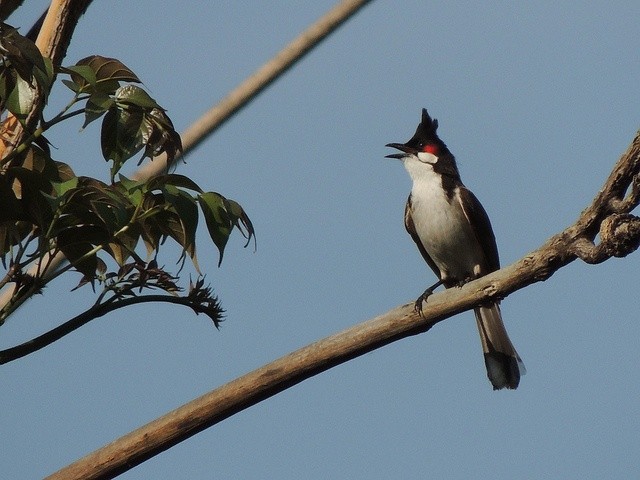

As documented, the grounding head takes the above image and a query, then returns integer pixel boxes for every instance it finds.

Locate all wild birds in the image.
[383,107,528,392]
